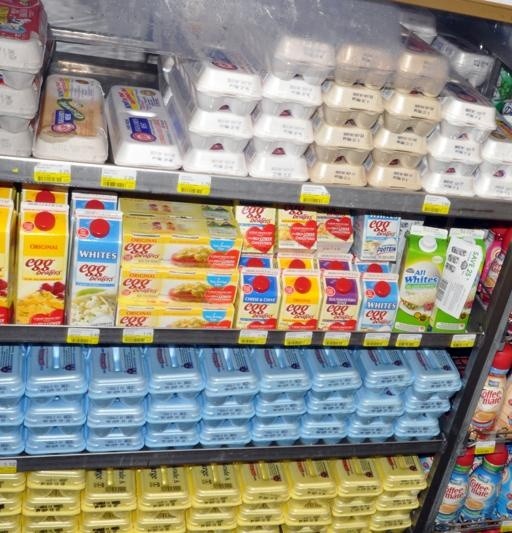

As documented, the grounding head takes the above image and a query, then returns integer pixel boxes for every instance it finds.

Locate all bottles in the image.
[420,225,512,529]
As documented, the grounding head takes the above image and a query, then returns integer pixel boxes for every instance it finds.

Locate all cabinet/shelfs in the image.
[0,0,511,533]
